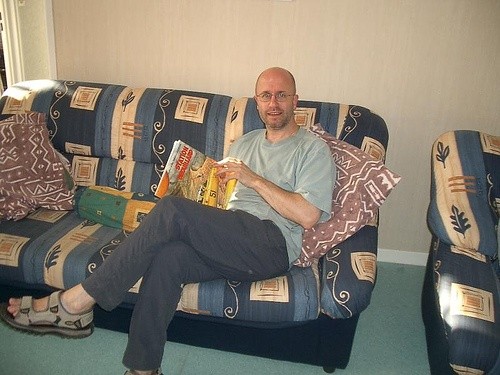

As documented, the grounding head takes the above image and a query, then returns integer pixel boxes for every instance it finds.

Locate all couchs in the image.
[422,128,500,375]
[0,78,388,375]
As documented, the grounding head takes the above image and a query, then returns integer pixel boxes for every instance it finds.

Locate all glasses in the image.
[255,91,294,102]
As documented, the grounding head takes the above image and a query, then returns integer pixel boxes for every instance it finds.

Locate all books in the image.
[155,142,237,210]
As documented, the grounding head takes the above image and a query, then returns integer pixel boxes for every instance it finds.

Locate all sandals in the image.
[0,290,94,339]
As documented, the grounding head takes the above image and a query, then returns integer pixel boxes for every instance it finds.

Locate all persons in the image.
[0,67,337,375]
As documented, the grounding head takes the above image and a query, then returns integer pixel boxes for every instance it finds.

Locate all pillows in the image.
[0,111,76,221]
[76,184,158,232]
[294,124,402,267]
